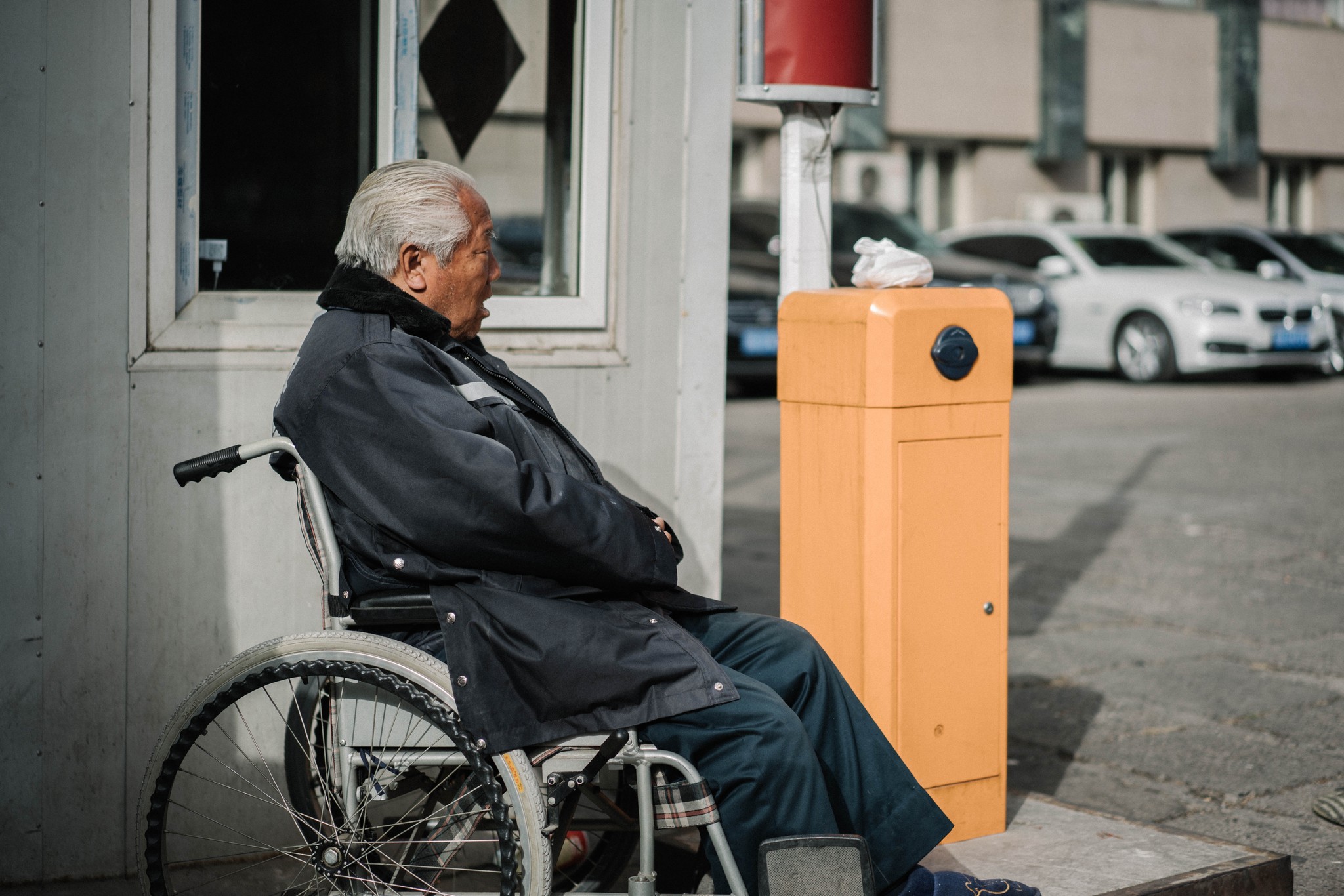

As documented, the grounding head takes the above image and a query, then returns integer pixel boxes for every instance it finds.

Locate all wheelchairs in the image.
[134,433,879,896]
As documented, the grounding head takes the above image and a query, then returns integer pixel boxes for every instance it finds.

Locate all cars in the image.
[1158,221,1344,381]
[928,215,1332,385]
[489,212,839,399]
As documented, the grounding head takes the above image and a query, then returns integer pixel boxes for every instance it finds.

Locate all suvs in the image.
[727,191,1062,385]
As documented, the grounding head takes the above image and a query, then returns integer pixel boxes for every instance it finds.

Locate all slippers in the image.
[900,870,1042,896]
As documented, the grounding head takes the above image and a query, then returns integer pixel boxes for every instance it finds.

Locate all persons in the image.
[272,160,1039,896]
